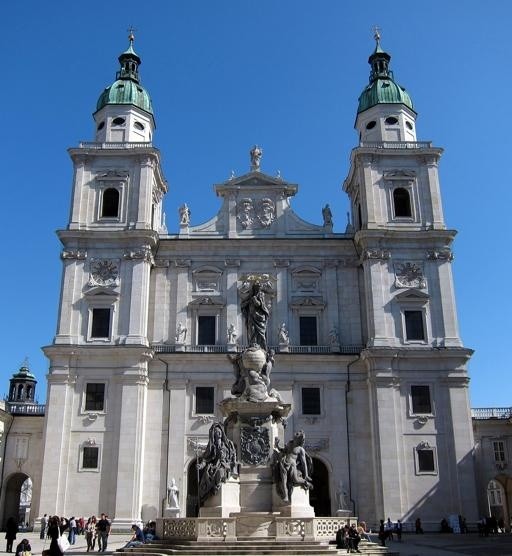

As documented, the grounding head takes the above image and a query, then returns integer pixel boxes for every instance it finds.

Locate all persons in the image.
[279,323,289,346]
[15,538,31,556]
[322,202,333,224]
[335,480,350,510]
[441,518,448,531]
[5,515,17,552]
[250,143,262,165]
[39,512,156,555]
[415,518,424,534]
[174,320,186,343]
[179,202,189,223]
[378,520,387,546]
[336,519,373,554]
[386,517,394,540]
[167,478,179,508]
[459,513,504,535]
[194,421,238,506]
[394,519,403,541]
[227,323,238,343]
[329,324,340,344]
[275,428,314,502]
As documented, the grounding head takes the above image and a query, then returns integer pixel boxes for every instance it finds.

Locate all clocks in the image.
[89,258,120,286]
[394,259,425,290]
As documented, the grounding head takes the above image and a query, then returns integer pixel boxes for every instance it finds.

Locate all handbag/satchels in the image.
[19,551,31,555]
[57,533,72,554]
